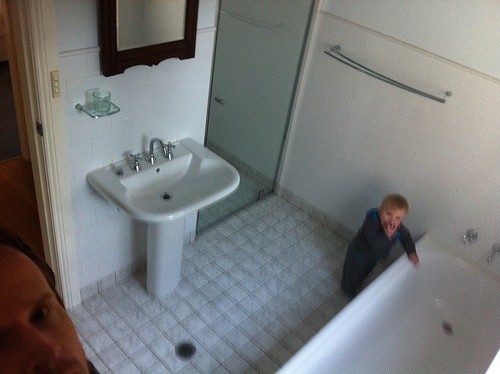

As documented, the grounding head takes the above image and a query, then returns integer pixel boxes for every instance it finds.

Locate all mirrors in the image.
[96,0,199,78]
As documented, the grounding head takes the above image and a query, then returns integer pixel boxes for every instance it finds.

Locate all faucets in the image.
[150,137,166,164]
[487,242,500,261]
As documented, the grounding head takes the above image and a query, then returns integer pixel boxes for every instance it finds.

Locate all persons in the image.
[0,228,101,374]
[342,194,418,298]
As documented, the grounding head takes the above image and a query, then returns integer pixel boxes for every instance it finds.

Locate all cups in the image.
[93,90,111,112]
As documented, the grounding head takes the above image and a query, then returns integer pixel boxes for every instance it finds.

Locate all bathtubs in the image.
[275,232,500,374]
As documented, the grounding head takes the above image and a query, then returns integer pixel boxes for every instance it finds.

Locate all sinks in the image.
[121,155,240,222]
